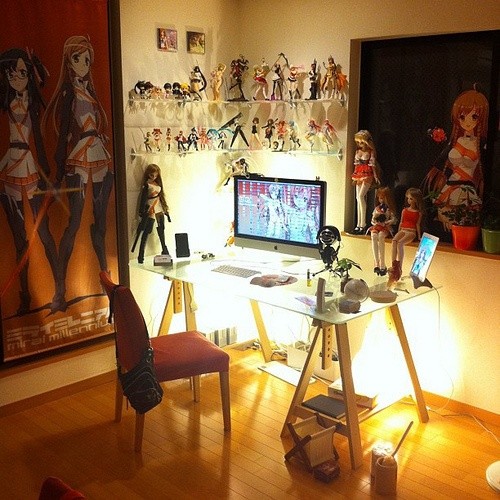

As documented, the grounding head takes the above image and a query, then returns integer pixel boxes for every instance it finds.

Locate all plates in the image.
[370,289,396,302]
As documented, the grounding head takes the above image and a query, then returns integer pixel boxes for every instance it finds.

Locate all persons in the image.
[131,164,172,264]
[387,188,425,279]
[129,51,347,152]
[370,187,397,275]
[350,129,380,235]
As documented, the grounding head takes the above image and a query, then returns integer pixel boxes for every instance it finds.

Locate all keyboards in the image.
[212,265,259,278]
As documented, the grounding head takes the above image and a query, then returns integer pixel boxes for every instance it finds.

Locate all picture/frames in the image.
[0,1,130,381]
[409,232,440,283]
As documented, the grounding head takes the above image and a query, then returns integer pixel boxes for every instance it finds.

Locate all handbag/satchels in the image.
[116,351,163,415]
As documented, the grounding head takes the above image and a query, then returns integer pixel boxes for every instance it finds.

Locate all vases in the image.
[451,222,482,251]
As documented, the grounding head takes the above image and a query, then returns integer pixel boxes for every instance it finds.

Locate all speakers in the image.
[174,233,190,257]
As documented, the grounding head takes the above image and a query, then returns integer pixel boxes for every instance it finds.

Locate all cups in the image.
[370,449,398,496]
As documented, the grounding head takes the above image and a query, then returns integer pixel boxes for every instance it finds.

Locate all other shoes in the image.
[380,266,387,276]
[355,225,369,235]
[374,266,380,276]
[349,227,360,234]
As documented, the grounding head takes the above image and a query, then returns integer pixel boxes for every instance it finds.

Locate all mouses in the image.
[262,279,278,287]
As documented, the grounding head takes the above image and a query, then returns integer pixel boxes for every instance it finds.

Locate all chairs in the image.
[98,270,232,452]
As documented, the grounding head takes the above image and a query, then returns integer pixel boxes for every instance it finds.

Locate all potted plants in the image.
[480,219,500,254]
[334,258,363,294]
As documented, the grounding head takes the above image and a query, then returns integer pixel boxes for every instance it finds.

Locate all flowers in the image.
[442,202,482,226]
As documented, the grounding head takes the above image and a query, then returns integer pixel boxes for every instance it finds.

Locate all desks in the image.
[128,246,443,471]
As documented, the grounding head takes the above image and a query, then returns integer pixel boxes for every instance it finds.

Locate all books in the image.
[301,394,346,419]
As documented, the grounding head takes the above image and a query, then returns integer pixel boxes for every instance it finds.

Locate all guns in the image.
[130,204,155,253]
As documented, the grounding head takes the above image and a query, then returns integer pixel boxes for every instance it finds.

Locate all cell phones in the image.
[277,275,289,283]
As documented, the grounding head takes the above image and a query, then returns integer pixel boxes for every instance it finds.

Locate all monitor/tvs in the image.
[233,174,327,260]
